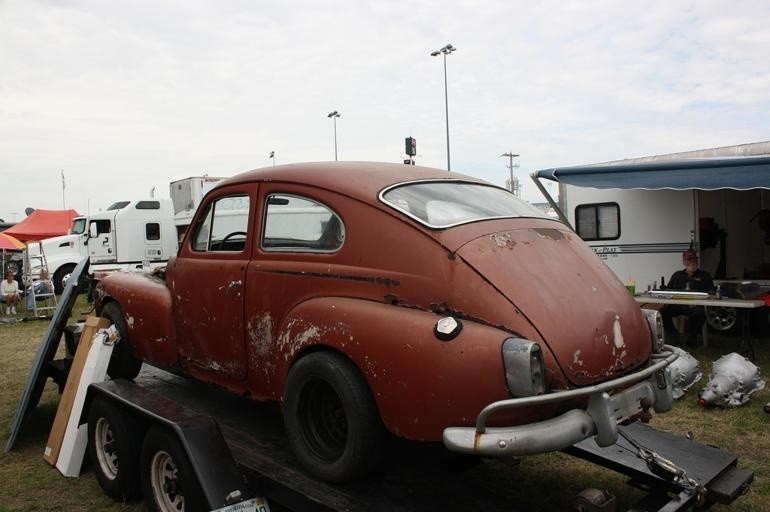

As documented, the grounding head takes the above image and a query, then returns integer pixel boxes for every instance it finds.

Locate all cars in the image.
[93,159,703,486]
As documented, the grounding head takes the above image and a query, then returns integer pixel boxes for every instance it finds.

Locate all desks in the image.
[633,292,765,363]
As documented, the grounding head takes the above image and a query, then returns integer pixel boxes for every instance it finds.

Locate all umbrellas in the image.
[0,232,26,279]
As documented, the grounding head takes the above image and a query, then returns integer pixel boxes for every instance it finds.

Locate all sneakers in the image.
[6,310,17,315]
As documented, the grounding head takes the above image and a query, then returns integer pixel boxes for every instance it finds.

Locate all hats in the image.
[683,251,698,259]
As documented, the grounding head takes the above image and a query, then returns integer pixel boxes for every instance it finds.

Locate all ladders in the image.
[22,240,58,322]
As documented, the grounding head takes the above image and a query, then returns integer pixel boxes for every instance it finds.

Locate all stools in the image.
[0,295,25,315]
[34,295,54,315]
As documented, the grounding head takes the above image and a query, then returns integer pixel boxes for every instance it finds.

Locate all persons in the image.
[659,250,715,351]
[26,269,55,311]
[0,271,22,316]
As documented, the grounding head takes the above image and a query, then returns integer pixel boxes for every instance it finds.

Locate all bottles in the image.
[652,280,657,289]
[715,284,722,300]
[659,275,666,289]
[647,279,652,291]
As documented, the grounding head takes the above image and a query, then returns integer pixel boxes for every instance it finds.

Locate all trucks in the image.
[21,212,90,301]
[83,175,336,304]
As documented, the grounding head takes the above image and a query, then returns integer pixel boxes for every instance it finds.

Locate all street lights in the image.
[429,43,457,172]
[270,151,277,166]
[328,111,343,161]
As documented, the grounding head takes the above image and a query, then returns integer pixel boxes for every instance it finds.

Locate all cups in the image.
[624,280,636,296]
